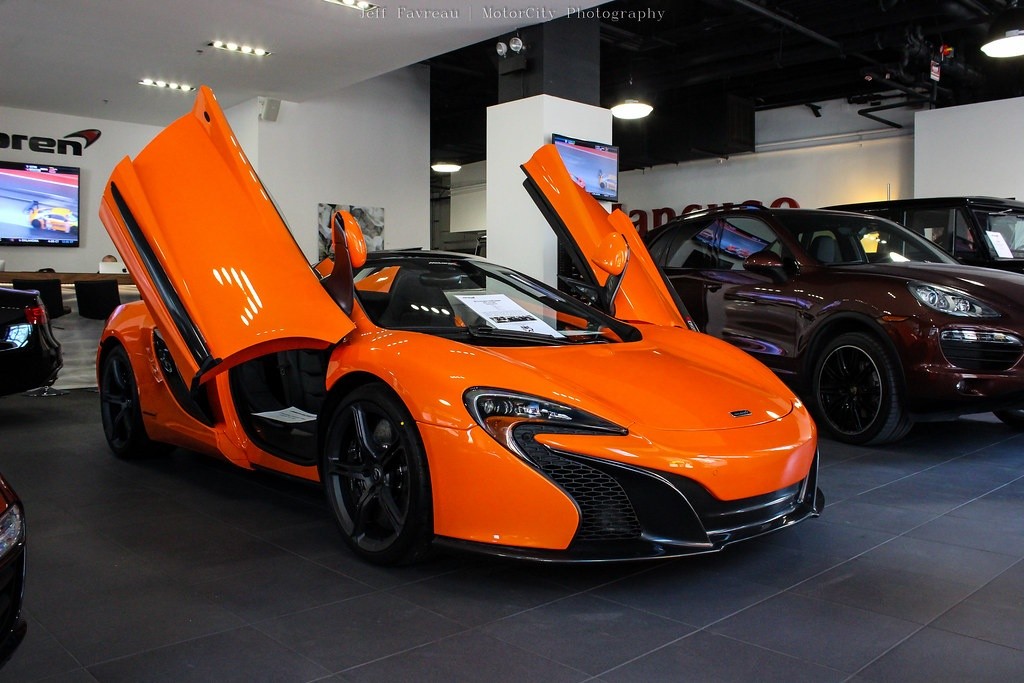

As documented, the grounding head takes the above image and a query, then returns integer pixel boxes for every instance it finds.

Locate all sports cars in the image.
[88,86,827,575]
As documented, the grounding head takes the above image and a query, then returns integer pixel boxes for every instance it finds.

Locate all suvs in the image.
[815,194,1024,273]
[638,206,1024,446]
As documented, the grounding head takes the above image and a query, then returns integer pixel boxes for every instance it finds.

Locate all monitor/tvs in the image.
[552,133,620,202]
[0,160,80,246]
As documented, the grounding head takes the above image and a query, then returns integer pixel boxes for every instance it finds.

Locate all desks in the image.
[0,271,136,285]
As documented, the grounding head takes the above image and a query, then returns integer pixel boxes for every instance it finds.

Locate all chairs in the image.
[74,281,121,321]
[383,267,455,328]
[13,278,73,331]
[809,235,835,265]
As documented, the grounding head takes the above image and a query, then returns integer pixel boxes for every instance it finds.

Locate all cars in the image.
[0,282,63,402]
[0,474,27,672]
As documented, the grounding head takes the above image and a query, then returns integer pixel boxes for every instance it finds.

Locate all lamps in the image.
[430,161,462,173]
[610,76,653,121]
[495,36,527,76]
[980,0,1024,58]
